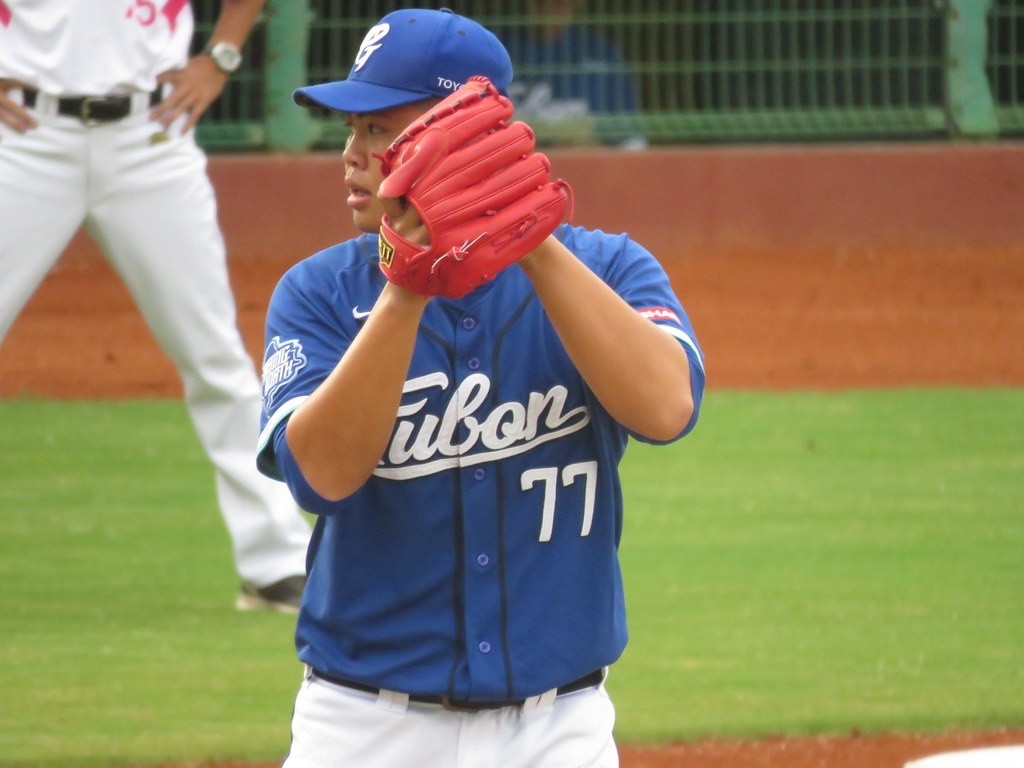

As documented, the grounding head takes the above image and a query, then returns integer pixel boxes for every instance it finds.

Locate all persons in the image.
[506,0,639,114]
[0,0,317,617]
[255,7,707,768]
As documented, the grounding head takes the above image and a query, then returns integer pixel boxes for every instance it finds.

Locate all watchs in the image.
[197,40,242,73]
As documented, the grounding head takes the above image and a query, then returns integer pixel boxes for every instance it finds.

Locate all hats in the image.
[294,7,514,114]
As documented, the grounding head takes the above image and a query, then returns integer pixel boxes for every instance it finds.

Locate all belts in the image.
[23,88,163,128]
[311,670,603,709]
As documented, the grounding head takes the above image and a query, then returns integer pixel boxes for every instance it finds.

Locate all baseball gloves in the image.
[366,74,579,295]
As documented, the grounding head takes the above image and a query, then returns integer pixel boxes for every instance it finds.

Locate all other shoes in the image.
[236,575,304,610]
